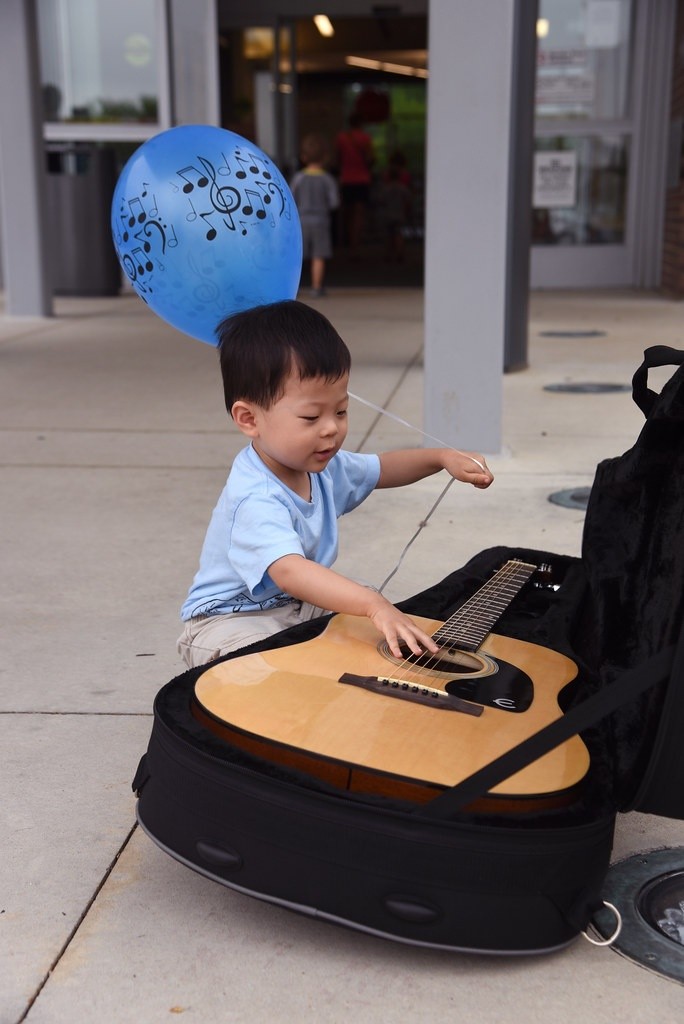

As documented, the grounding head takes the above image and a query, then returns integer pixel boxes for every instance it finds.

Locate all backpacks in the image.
[538,345,684,821]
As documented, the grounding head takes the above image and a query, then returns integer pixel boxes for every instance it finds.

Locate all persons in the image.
[336,115,374,257]
[291,134,339,297]
[377,152,412,205]
[177,300,495,667]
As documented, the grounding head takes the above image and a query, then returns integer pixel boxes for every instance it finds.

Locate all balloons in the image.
[112,125,302,343]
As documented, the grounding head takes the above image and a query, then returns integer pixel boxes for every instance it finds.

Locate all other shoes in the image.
[309,288,324,298]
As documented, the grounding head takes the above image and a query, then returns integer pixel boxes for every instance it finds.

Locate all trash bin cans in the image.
[45,142,122,298]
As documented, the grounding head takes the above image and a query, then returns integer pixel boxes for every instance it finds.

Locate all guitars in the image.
[189,557,591,814]
